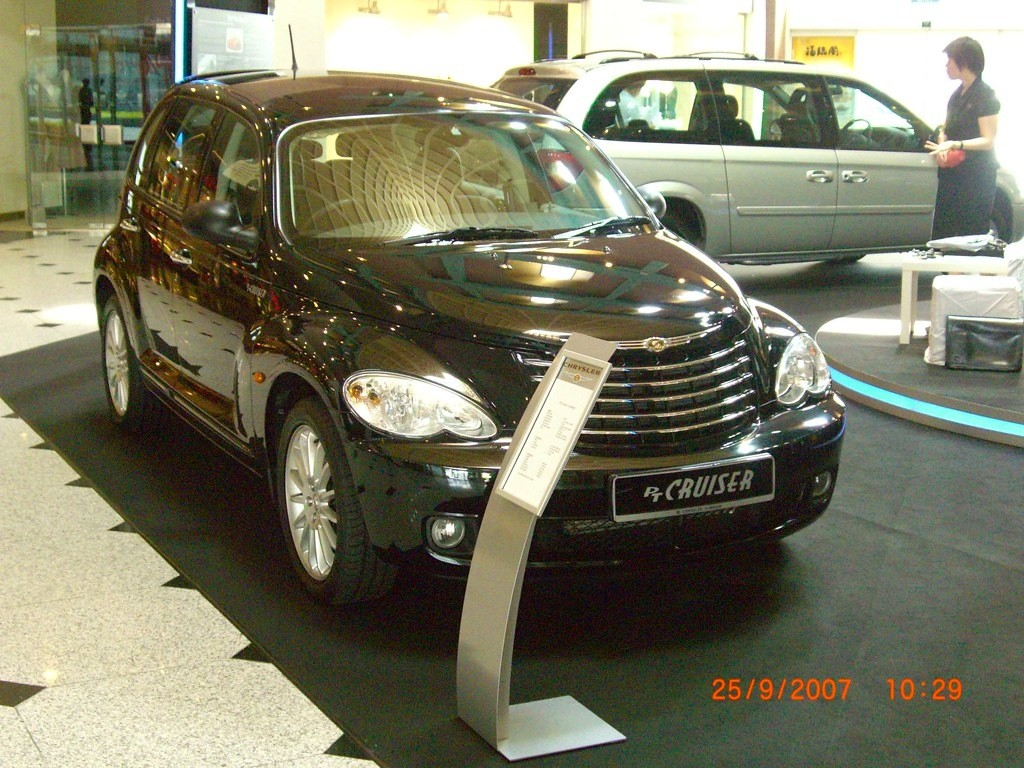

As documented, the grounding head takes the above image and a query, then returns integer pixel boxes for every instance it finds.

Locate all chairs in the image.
[778,90,817,148]
[285,125,399,234]
[699,92,755,143]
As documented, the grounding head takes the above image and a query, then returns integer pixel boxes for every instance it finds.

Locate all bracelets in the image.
[960,140,964,149]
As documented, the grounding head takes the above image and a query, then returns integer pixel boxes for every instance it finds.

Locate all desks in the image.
[854,251,1024,344]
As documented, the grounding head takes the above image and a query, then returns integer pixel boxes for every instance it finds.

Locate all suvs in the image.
[94,67,847,612]
[485,47,1022,264]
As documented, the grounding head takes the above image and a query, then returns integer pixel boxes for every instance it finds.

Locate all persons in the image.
[923,37,1003,276]
[79,77,94,125]
